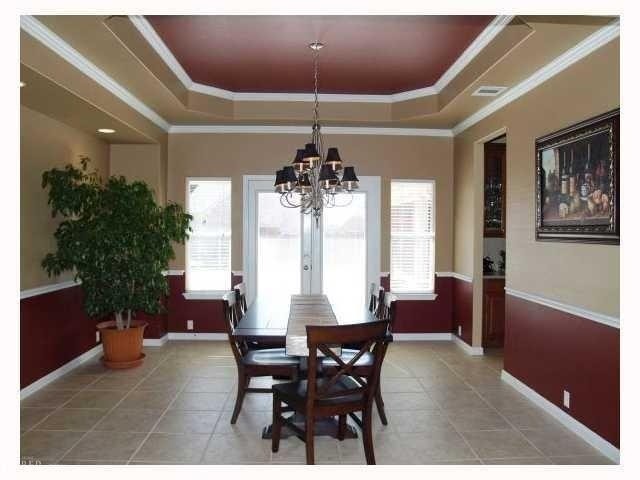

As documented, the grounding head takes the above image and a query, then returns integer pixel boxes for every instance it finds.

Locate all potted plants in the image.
[40,152,194,369]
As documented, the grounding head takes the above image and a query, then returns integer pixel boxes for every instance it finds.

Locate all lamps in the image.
[273,42,360,227]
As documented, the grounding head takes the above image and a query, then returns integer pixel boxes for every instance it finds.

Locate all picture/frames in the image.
[535,108,619,245]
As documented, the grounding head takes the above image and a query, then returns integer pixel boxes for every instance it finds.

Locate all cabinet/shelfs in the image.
[482,278,505,352]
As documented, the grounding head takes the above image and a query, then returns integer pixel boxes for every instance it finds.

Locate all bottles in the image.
[558,142,595,197]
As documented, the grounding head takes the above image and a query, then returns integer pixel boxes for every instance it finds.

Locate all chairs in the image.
[222,283,300,424]
[322,283,398,425]
[272,319,388,464]
